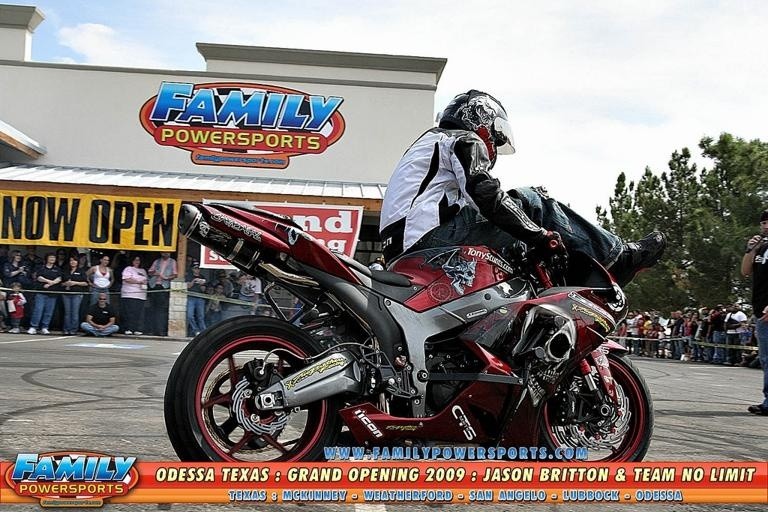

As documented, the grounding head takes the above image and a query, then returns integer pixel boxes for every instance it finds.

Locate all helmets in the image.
[442,90,515,154]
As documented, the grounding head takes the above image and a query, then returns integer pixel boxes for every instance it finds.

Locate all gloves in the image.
[544,231,568,261]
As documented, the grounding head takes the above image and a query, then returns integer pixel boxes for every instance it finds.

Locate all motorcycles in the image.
[163,183,655,463]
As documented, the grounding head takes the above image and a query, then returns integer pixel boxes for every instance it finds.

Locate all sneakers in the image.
[8,327,50,334]
[748,404,768,416]
[608,231,667,289]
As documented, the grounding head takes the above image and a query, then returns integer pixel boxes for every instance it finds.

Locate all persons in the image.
[379,89,667,287]
[1,242,768,376]
[741,209,768,417]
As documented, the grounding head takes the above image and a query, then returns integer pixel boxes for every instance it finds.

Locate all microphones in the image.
[745,230,767,253]
